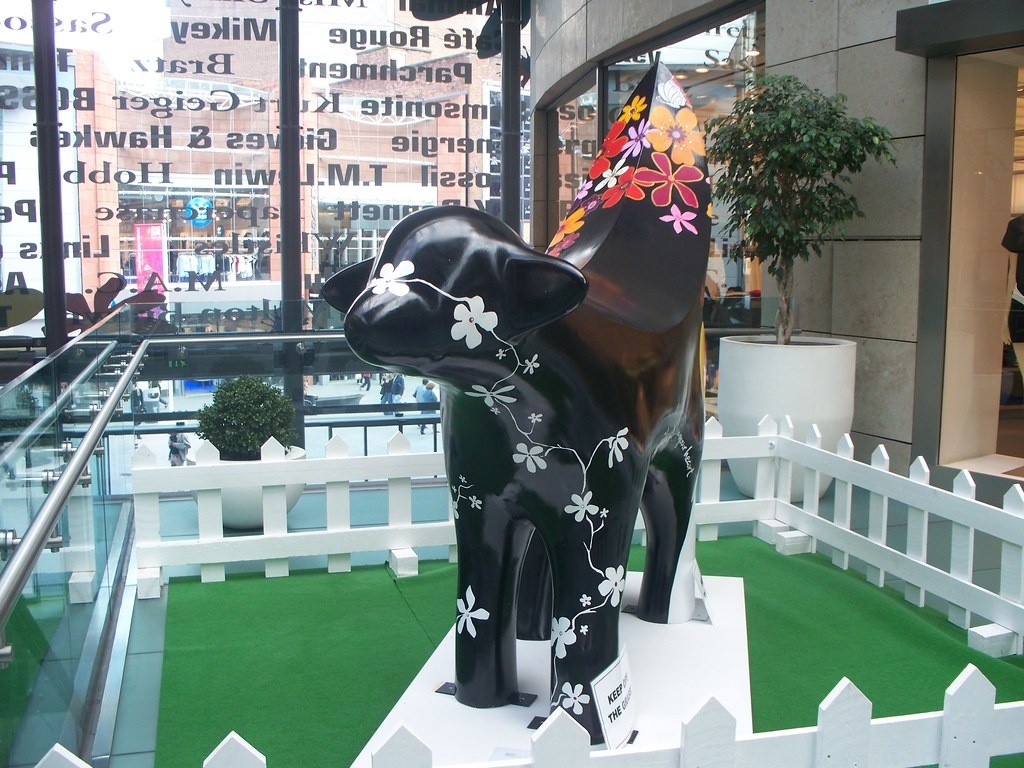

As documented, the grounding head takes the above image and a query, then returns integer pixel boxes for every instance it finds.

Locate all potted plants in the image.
[701,65,900,502]
[186,373,307,529]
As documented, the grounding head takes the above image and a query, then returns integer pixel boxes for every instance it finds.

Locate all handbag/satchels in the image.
[412,386,419,398]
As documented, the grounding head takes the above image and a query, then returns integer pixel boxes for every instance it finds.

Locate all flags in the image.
[134,224,166,321]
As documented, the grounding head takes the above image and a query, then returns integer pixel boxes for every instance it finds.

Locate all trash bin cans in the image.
[305,394,318,415]
[142,387,160,424]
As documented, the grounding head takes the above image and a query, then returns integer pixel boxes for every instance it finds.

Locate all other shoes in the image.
[421,431,425,434]
[165,402,168,408]
[137,435,142,439]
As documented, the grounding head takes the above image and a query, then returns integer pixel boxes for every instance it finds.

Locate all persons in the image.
[1000,215,1023,375]
[360,373,372,392]
[413,379,429,427]
[168,432,192,466]
[132,383,144,439]
[420,382,438,434]
[355,374,362,383]
[148,380,168,408]
[379,373,405,415]
[303,381,315,406]
